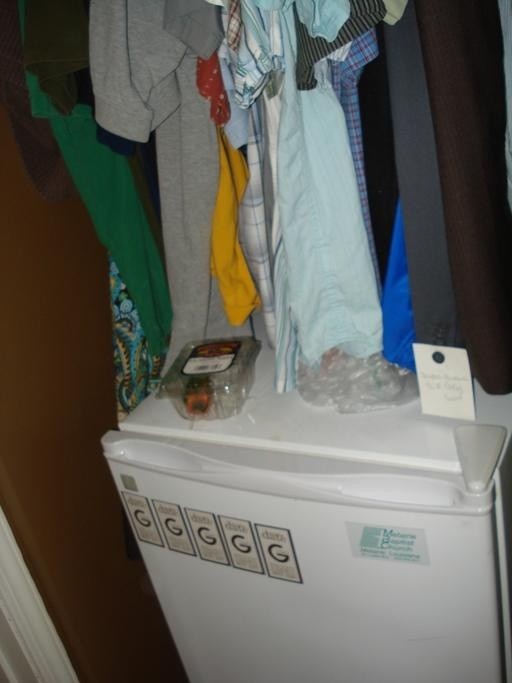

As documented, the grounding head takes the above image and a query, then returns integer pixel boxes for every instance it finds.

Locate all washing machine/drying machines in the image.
[99,346,512,682]
